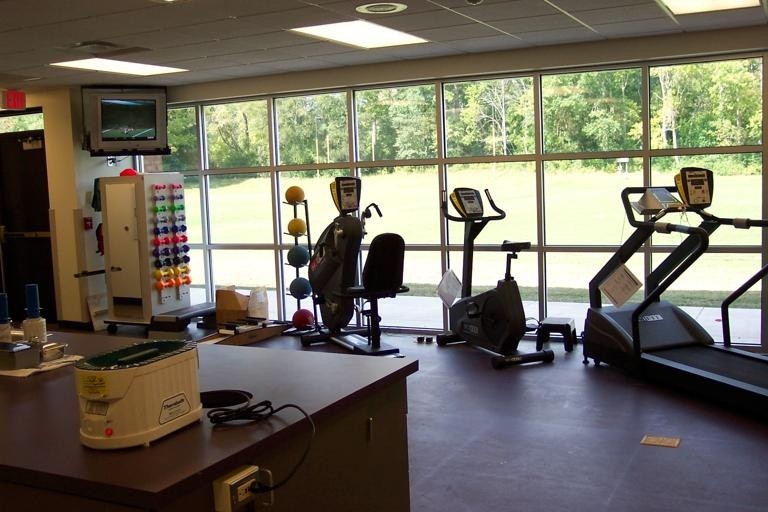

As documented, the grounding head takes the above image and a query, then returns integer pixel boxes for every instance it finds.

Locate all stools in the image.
[536,318,577,352]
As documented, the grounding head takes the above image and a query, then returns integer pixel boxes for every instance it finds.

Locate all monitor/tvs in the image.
[90,93,167,150]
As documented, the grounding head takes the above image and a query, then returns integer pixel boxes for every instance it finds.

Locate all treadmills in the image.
[583,165,768,422]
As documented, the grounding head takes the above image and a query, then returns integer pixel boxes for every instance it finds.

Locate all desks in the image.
[0,331,418,512]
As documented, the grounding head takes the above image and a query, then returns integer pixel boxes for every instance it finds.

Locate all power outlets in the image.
[213,465,275,512]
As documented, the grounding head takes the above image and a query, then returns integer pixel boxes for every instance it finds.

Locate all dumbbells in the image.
[151,183,191,290]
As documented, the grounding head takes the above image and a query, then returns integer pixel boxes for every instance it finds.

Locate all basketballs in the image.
[292,310,313,329]
[286,187,305,204]
[287,245,309,268]
[289,218,307,236]
[289,277,311,298]
